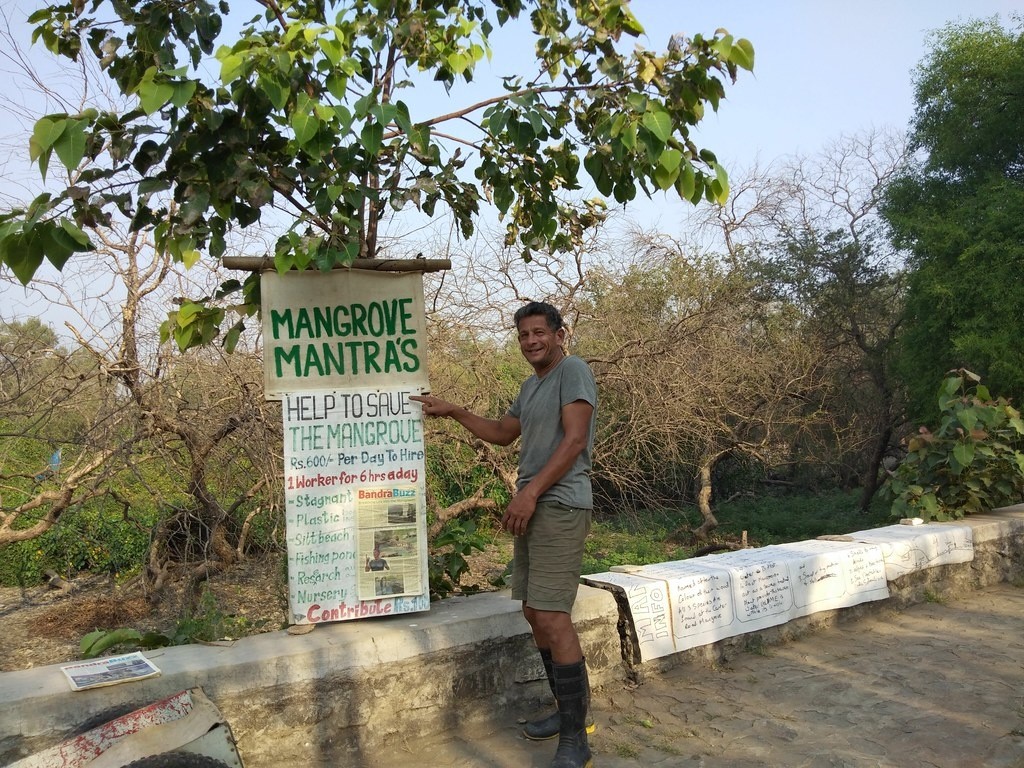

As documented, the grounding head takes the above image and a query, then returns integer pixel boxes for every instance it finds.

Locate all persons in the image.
[409,302,599,768]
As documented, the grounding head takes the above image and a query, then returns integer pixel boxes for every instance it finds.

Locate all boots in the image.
[551,656,595,768]
[523,648,595,740]
